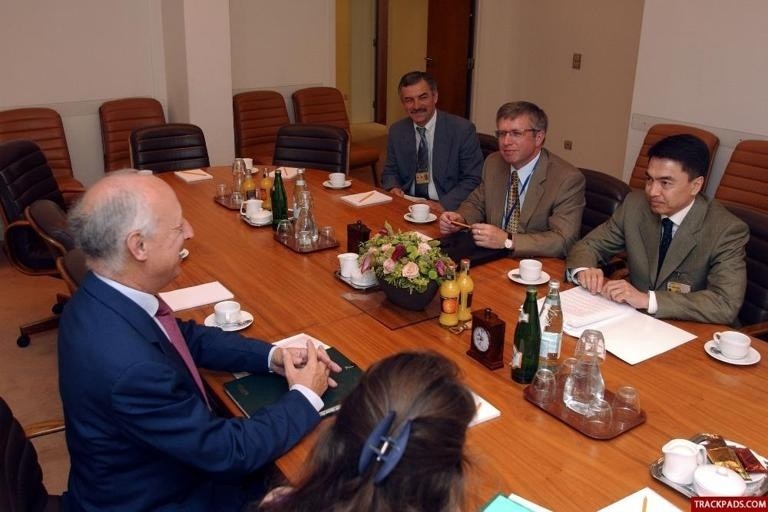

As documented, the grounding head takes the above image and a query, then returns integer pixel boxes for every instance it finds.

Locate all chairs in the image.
[575,164,633,278]
[712,138,767,216]
[232,89,291,166]
[291,83,382,189]
[97,96,166,178]
[271,124,352,180]
[475,130,500,164]
[0,105,92,348]
[598,199,767,346]
[624,123,720,200]
[1,393,78,509]
[125,120,210,182]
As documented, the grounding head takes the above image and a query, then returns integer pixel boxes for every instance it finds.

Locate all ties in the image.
[504,171,521,234]
[154,294,213,413]
[414,126,431,200]
[656,217,674,280]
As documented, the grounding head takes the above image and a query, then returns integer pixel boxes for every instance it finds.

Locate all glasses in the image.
[495,128,541,138]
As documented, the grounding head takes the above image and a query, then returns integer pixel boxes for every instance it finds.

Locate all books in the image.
[432,228,509,272]
[224,346,367,428]
[525,285,698,365]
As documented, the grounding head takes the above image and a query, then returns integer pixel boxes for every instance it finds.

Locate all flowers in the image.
[351,216,462,299]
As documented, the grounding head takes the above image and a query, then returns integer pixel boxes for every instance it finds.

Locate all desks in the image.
[123,163,768,512]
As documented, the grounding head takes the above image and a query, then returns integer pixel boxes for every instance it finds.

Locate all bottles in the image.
[511,280,562,383]
[240,167,308,230]
[438,259,475,327]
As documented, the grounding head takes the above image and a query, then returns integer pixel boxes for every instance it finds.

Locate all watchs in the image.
[504,233,513,250]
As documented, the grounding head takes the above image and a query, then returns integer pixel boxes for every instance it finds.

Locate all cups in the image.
[214,155,245,206]
[529,359,643,435]
[562,330,605,416]
[336,252,376,286]
[213,301,242,324]
[660,438,706,485]
[518,258,542,281]
[408,204,429,220]
[713,331,751,358]
[275,191,337,247]
[328,172,345,186]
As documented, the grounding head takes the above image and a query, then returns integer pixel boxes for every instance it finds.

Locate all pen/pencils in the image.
[284,168,288,176]
[359,192,374,203]
[182,171,207,176]
[642,496,647,512]
[452,221,470,228]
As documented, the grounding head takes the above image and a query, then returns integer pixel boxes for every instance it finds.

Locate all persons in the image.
[381,71,484,213]
[560,132,751,328]
[255,350,477,512]
[56,169,342,512]
[440,100,586,258]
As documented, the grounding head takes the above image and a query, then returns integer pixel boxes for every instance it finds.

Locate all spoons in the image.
[215,319,251,328]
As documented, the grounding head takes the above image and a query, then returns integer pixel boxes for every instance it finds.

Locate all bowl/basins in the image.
[694,464,744,497]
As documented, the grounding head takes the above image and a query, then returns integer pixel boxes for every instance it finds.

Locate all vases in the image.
[369,265,440,313]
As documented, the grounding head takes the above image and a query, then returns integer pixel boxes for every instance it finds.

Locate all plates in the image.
[508,267,550,286]
[403,212,437,224]
[707,440,765,484]
[321,180,351,189]
[203,310,254,333]
[703,339,760,367]
